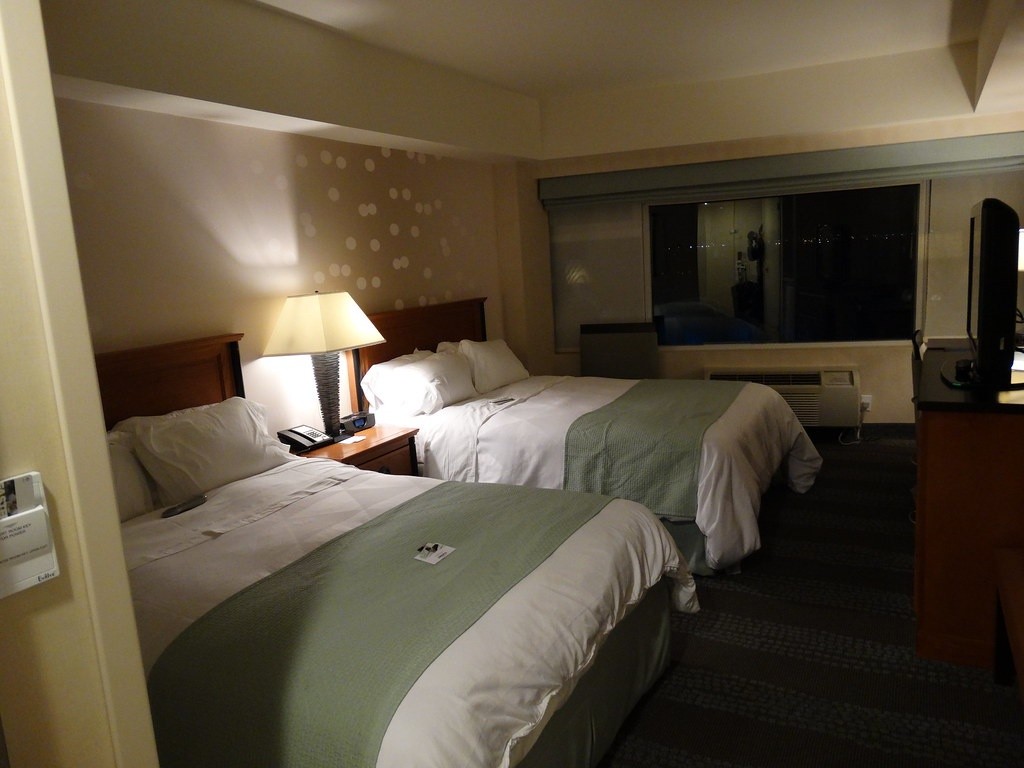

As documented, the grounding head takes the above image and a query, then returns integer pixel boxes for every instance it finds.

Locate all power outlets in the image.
[862,395,872,411]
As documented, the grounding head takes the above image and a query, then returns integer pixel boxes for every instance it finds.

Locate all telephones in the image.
[277,425,333,455]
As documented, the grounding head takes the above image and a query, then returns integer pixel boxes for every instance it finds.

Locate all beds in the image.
[94,334,701,767]
[358,297,823,576]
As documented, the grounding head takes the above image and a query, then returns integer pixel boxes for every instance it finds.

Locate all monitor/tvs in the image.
[937,198,1024,390]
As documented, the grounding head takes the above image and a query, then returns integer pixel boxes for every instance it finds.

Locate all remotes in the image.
[160,495,207,519]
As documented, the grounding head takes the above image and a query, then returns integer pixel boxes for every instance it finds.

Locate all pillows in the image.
[117,396,299,504]
[459,340,530,393]
[106,429,155,525]
[360,348,437,413]
[373,349,480,417]
[437,341,461,354]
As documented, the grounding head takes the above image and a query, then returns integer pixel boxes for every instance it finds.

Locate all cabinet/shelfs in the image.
[916,339,1024,672]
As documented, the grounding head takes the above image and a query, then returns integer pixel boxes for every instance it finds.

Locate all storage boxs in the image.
[703,364,861,428]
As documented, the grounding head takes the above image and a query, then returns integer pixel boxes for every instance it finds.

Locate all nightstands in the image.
[299,424,420,476]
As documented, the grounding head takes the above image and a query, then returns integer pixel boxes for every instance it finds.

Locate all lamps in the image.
[262,290,388,436]
